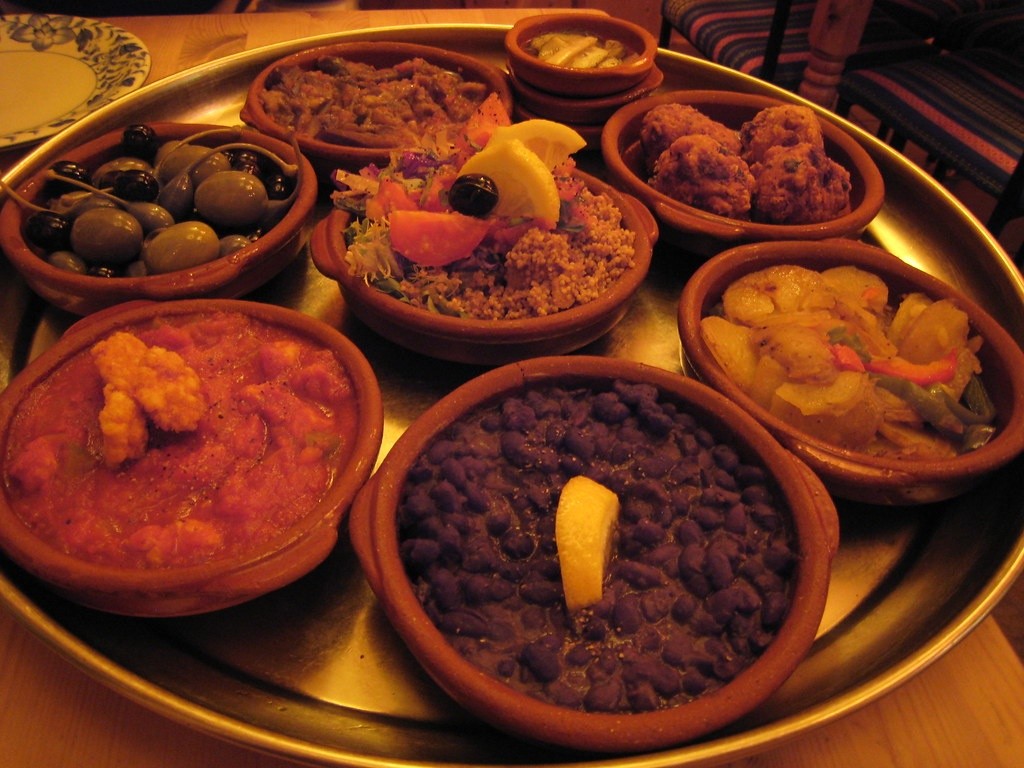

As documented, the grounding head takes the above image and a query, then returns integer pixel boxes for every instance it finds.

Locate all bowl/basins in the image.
[350,356,840,755]
[0,298,383,618]
[239,43,513,197]
[0,120,316,316]
[503,13,657,96]
[677,237,1023,505]
[310,170,659,363]
[602,91,886,257]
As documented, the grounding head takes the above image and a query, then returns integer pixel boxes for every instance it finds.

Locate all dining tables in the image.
[0,15,1024,768]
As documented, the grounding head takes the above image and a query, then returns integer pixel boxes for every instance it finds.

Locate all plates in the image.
[503,58,663,125]
[0,13,150,148]
[512,100,606,152]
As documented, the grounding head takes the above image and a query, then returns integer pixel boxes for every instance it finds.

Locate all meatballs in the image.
[641,101,852,225]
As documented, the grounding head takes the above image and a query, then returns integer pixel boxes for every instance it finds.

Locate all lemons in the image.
[483,118,587,173]
[456,140,560,221]
[554,475,621,611]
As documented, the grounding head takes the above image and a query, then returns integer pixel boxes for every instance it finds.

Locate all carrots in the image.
[366,172,494,268]
